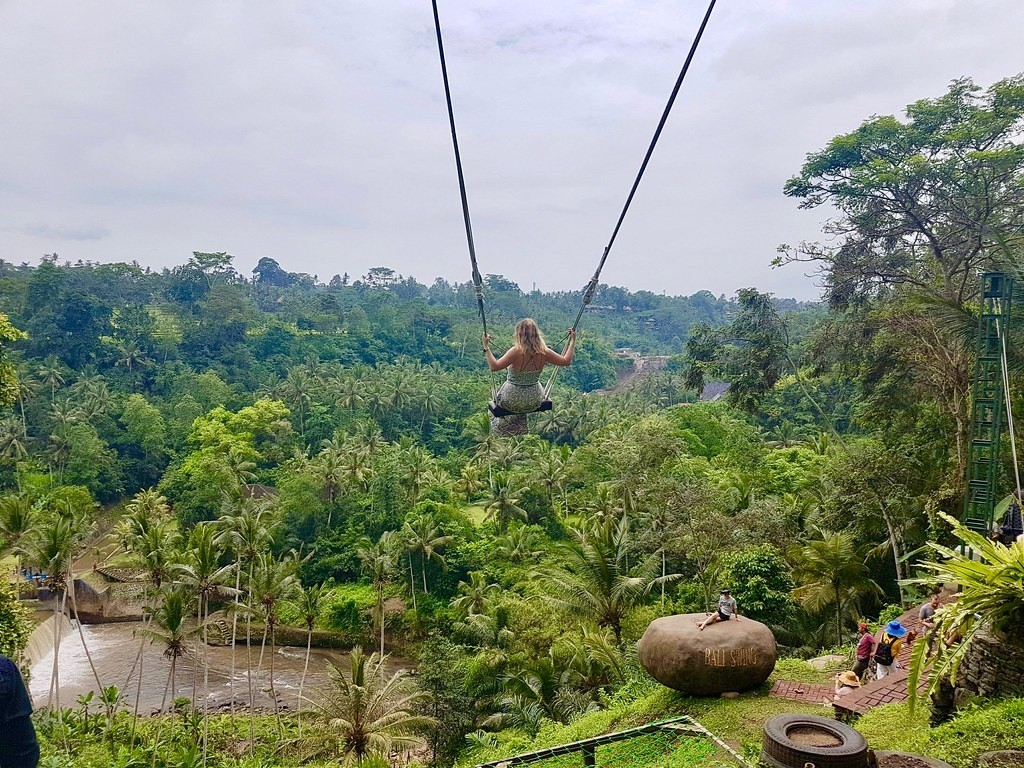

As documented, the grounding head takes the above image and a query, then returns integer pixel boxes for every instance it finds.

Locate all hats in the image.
[883,620,907,639]
[839,671,862,687]
[720,587,731,594]
[859,622,868,632]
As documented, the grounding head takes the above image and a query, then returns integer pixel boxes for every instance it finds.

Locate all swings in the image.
[430,1,723,418]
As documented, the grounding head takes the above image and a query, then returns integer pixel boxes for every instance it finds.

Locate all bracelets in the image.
[483,348,489,353]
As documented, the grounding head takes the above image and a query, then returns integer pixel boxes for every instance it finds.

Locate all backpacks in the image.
[874,633,898,666]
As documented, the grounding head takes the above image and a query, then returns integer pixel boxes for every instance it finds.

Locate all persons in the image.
[919,594,945,662]
[986,522,1007,567]
[835,670,861,700]
[877,620,907,680]
[851,623,876,681]
[0,654,40,768]
[696,587,741,630]
[483,318,576,439]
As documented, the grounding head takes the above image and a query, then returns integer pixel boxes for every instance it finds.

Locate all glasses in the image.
[724,593,731,596]
